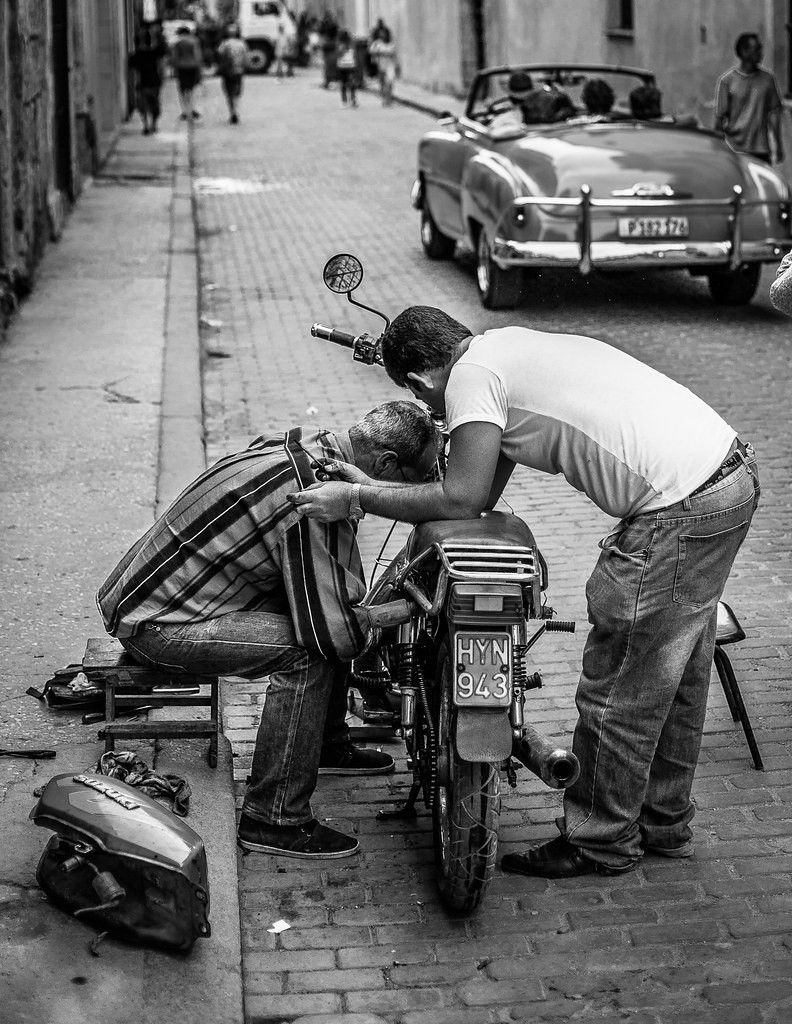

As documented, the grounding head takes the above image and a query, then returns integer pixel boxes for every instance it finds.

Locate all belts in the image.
[685,438,748,496]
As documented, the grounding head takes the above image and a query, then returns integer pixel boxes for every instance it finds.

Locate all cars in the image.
[410,59,792,315]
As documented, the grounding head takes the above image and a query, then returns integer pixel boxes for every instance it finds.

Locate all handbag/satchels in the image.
[215,52,233,76]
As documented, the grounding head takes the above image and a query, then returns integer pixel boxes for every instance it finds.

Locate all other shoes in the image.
[239,811,359,859]
[229,114,239,125]
[180,110,200,121]
[141,127,159,137]
[316,734,394,774]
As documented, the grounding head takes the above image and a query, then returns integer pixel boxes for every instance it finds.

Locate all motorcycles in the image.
[307,250,581,924]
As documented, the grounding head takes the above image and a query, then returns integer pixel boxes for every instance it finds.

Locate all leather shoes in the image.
[502,836,602,878]
[641,839,695,857]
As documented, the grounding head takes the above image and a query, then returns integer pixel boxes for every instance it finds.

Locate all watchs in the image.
[348,483,366,520]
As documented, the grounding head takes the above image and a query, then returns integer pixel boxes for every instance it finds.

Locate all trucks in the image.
[160,0,298,77]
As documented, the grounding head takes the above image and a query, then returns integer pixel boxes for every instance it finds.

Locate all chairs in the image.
[713,600,765,770]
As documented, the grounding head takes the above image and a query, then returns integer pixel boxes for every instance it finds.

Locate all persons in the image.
[94,401,445,861]
[488,73,535,129]
[714,30,785,167]
[629,87,664,119]
[127,9,402,137]
[581,78,617,116]
[287,305,761,880]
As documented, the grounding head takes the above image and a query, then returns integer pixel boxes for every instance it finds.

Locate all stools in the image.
[82,637,220,773]
